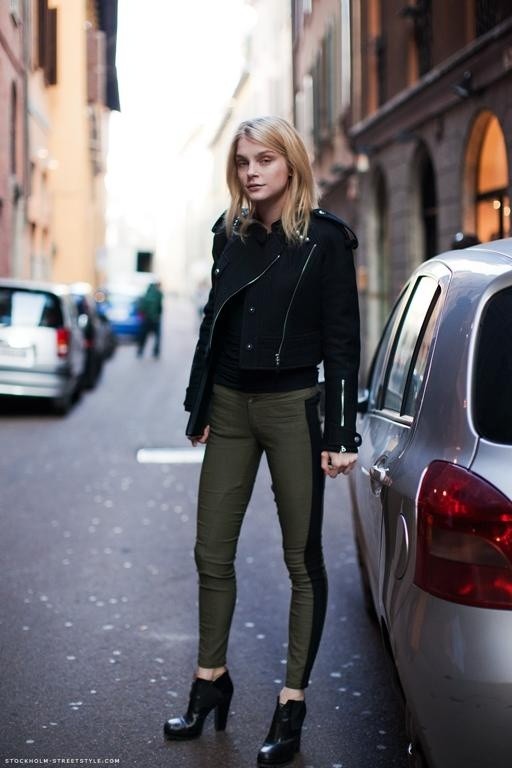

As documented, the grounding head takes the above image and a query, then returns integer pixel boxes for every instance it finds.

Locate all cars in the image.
[0,278,150,416]
[347,237,512,768]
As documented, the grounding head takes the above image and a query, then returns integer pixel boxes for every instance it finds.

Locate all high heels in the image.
[163,668,234,739]
[256,694,308,765]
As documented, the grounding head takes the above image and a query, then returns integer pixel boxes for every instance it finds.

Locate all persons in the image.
[163,118,362,767]
[137,278,165,358]
